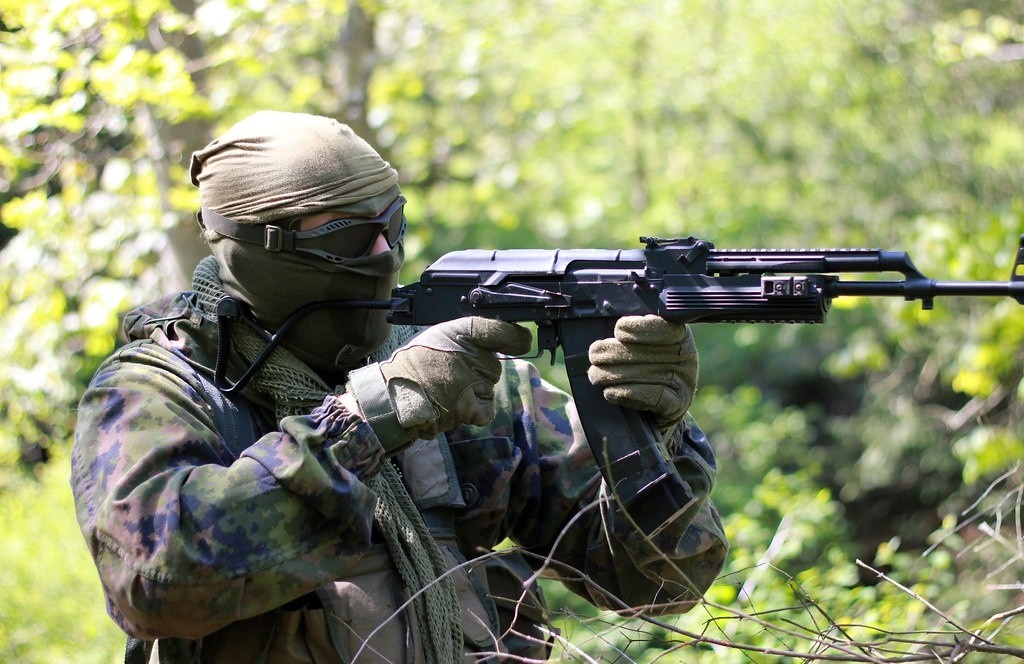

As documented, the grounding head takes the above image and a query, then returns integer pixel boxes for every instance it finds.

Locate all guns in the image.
[216,235,1023,530]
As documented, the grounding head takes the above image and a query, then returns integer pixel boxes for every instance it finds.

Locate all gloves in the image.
[345,315,532,457]
[588,315,698,433]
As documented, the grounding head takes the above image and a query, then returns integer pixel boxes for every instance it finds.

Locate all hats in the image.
[192,110,398,243]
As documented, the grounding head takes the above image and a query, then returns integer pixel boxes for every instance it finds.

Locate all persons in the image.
[70,110,730,664]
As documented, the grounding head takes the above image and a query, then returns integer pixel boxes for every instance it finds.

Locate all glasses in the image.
[201,196,407,263]
